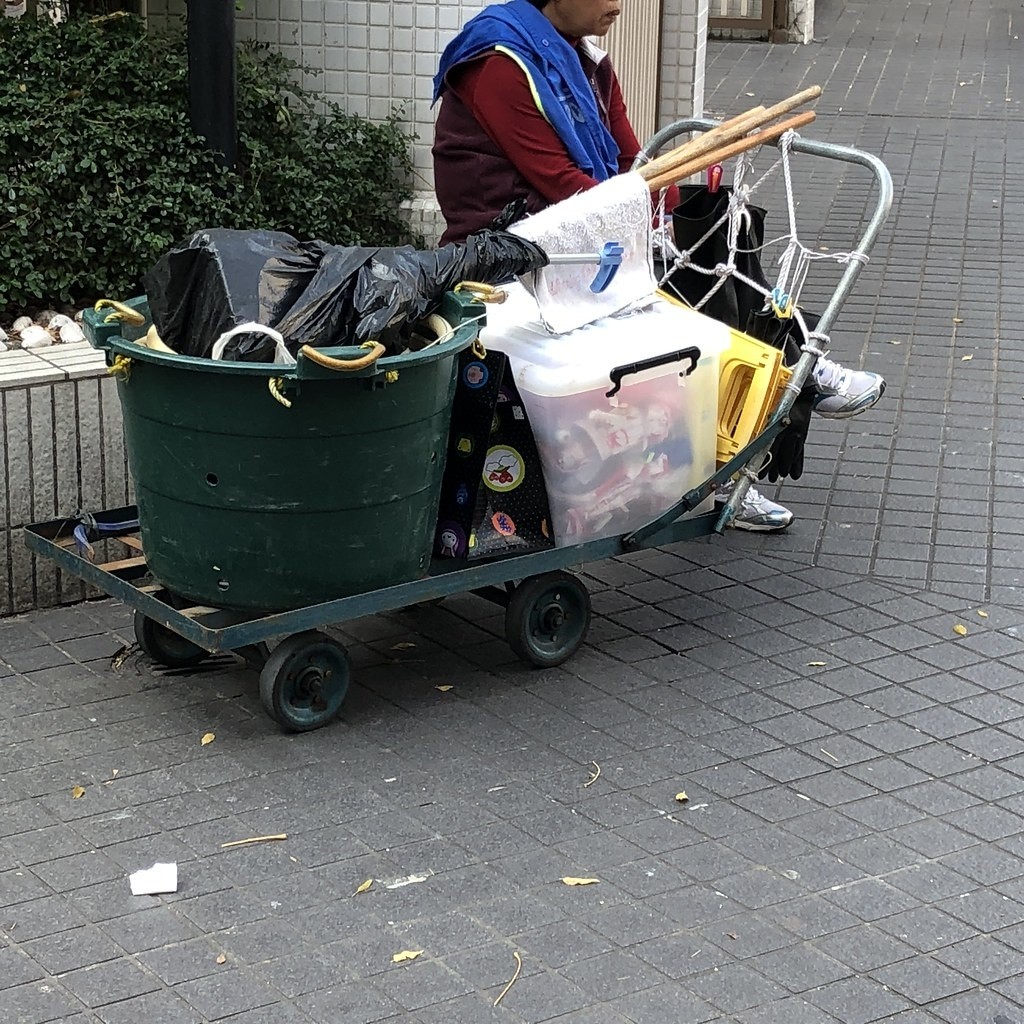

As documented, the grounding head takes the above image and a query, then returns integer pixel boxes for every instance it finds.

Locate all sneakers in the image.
[798,349,887,420]
[715,477,795,530]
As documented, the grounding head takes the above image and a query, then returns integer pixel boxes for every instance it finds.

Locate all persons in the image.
[428,0,885,532]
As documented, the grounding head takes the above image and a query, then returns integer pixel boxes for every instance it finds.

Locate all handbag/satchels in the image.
[433,345,555,563]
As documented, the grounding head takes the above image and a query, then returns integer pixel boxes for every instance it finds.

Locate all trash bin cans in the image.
[79,284,487,614]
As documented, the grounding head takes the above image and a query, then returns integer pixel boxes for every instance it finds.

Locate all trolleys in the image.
[18,118,896,733]
